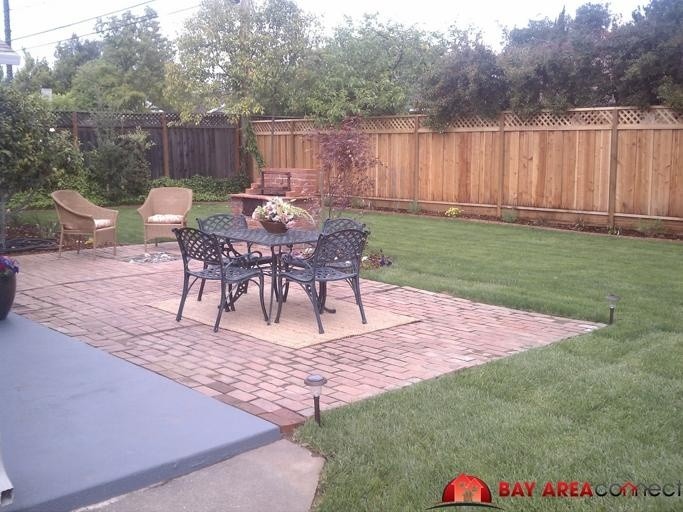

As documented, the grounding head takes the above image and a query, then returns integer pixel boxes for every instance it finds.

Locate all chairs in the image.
[51,190,117,260]
[172,227,269,333]
[274,228,371,333]
[195,215,279,304]
[136,187,192,252]
[283,218,366,309]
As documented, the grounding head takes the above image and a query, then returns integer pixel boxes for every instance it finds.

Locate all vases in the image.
[0,273,16,322]
[260,220,288,233]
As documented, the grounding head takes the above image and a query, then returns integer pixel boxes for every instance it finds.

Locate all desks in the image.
[199,229,344,325]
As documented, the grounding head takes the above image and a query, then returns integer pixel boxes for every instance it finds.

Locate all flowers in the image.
[252,196,317,229]
[0,254,19,278]
